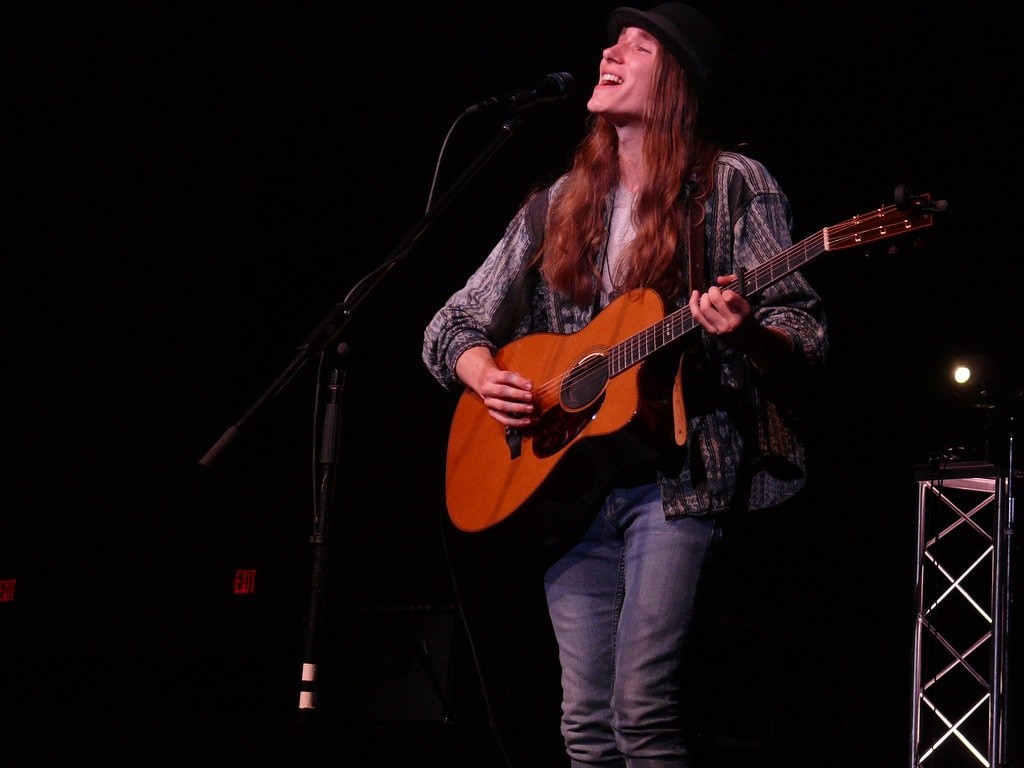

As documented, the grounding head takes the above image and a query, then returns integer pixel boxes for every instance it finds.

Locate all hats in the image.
[606,2,727,96]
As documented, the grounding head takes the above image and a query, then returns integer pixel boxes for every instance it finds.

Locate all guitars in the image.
[442,181,941,559]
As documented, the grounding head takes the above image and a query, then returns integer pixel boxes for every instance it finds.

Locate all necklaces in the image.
[606,253,628,289]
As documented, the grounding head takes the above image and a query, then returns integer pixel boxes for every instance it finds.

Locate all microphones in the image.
[465,72,573,116]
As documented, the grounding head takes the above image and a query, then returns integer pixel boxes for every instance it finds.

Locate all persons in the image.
[421,3,827,768]
